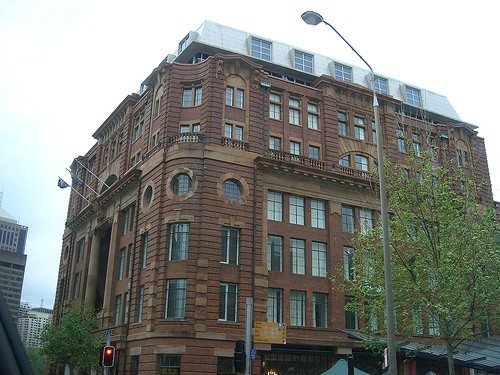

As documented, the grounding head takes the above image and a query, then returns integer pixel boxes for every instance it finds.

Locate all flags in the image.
[57,177,70,189]
[70,173,84,188]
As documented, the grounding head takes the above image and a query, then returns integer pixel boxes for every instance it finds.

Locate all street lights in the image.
[302,10,401,375]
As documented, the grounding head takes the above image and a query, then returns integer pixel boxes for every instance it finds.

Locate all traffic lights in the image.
[102,345,115,369]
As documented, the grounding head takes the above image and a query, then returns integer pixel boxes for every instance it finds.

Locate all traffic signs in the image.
[253,320,287,344]
